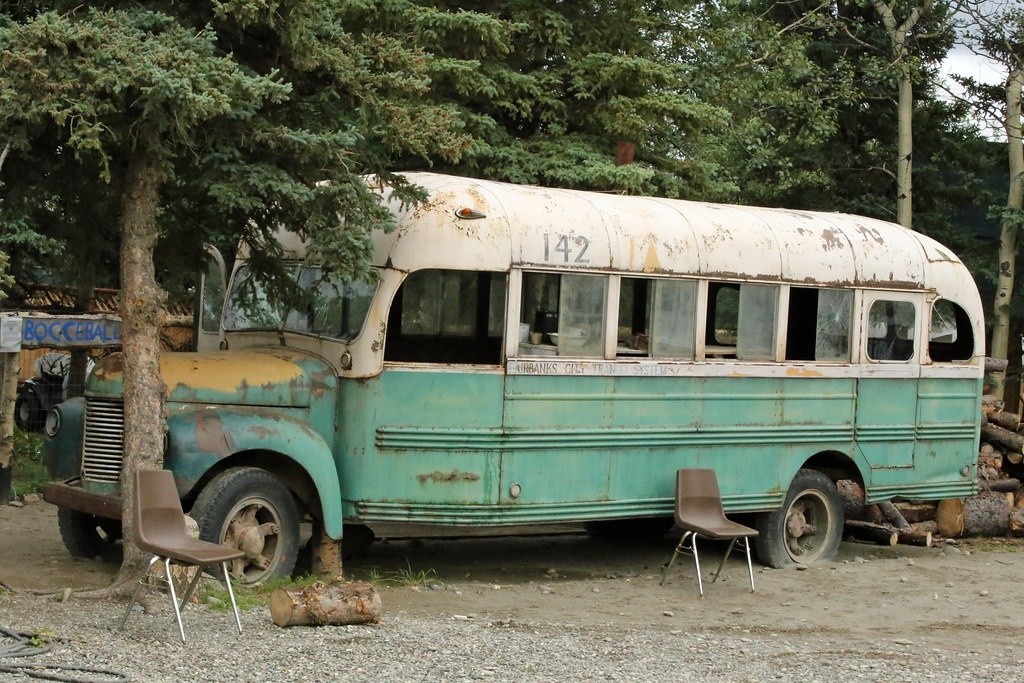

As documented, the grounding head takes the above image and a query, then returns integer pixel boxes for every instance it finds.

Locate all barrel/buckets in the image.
[519,322,530,343]
[530,331,543,345]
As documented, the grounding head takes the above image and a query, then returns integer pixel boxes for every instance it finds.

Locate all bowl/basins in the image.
[546,332,588,347]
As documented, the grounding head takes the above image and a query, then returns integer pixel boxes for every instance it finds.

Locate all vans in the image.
[42,170,986,593]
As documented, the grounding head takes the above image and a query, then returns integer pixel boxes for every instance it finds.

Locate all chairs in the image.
[118,468,245,644]
[659,468,759,599]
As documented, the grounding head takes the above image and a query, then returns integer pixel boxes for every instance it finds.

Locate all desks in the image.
[612,342,737,359]
